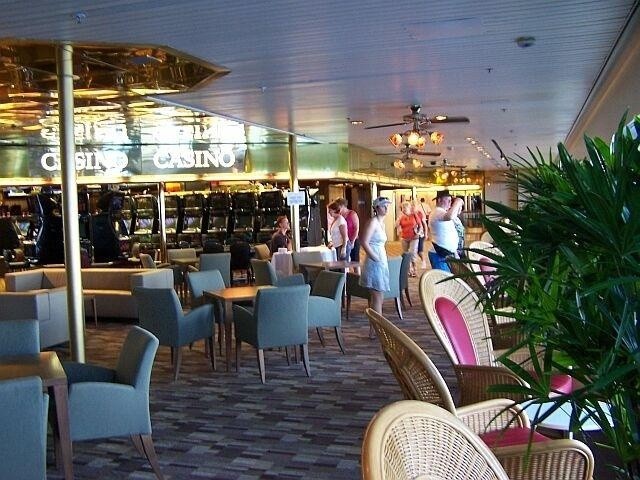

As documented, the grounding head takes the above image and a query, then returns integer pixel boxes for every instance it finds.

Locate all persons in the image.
[359,196,393,341]
[451,197,464,259]
[326,202,351,274]
[231,223,251,278]
[417,198,431,243]
[334,197,360,274]
[427,189,465,260]
[270,214,293,255]
[130,236,142,258]
[392,201,422,277]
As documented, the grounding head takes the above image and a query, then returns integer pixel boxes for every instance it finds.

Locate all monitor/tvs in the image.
[17,219,29,237]
[261,213,280,228]
[164,217,176,233]
[235,213,254,230]
[112,219,129,240]
[210,216,227,232]
[134,216,153,234]
[183,216,203,233]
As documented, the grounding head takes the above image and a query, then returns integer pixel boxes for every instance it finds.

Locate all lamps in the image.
[451,171,471,184]
[388,129,444,149]
[392,153,424,169]
[432,166,460,185]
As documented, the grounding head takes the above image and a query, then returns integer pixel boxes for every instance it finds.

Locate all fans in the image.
[362,104,469,130]
[375,143,442,156]
[433,159,467,169]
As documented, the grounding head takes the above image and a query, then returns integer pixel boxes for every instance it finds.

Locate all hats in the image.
[373,196,392,208]
[432,190,451,201]
